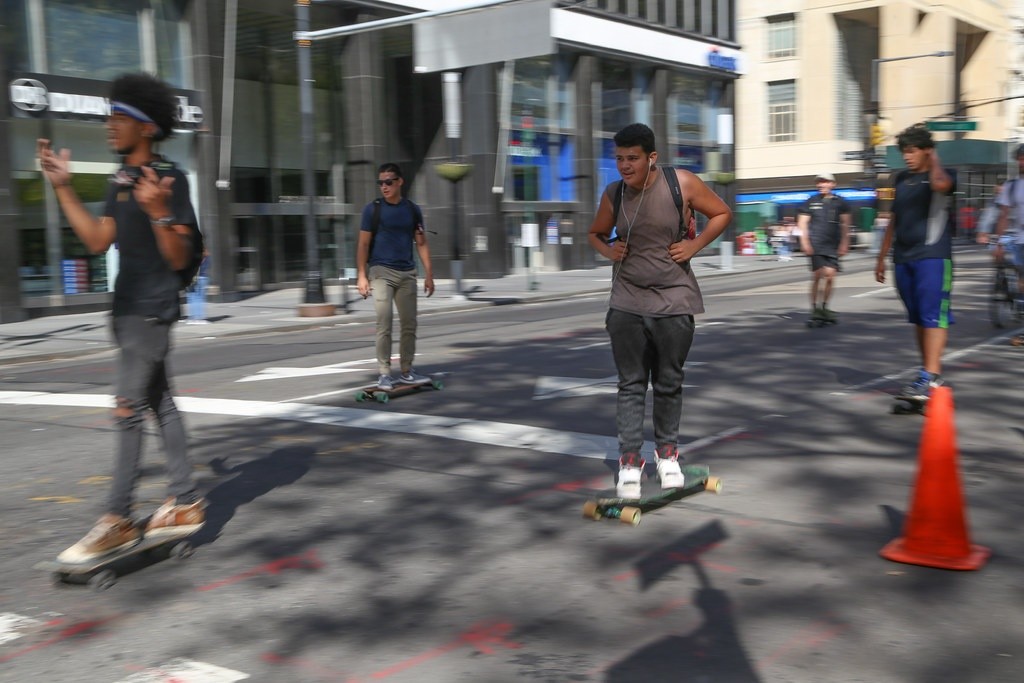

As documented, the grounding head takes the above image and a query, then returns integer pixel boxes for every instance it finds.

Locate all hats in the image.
[814,173,835,186]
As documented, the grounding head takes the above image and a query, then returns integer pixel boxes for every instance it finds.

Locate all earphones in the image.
[648,158,652,167]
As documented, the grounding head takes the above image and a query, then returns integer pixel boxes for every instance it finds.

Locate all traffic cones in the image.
[875,390,992,571]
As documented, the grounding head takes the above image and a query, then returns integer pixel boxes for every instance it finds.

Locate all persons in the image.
[36,72,208,564]
[586,124,735,500]
[355,163,435,389]
[794,172,852,319]
[876,129,962,402]
[976,145,1024,339]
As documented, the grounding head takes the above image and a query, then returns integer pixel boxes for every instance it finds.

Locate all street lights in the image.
[867,50,952,184]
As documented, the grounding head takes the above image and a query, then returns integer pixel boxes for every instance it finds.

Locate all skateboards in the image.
[355,373,443,403]
[893,376,946,415]
[582,463,723,527]
[805,318,840,326]
[35,520,210,592]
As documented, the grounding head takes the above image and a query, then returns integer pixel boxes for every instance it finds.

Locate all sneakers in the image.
[399,371,430,385]
[653,446,683,488]
[378,373,404,390]
[617,453,647,500]
[58,514,141,567]
[901,370,946,400]
[136,499,205,536]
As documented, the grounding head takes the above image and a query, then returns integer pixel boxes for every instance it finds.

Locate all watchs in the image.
[150,216,177,226]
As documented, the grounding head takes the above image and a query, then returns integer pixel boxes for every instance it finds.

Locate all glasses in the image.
[376,177,399,187]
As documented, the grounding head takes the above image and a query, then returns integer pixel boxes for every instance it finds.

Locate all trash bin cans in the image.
[234,247,263,292]
[322,279,348,314]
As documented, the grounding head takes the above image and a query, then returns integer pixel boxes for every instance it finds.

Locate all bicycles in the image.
[984,235,1017,329]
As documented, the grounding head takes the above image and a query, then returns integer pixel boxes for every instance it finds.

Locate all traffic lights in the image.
[869,125,883,147]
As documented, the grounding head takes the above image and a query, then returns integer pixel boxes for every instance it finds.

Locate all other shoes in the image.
[812,306,840,322]
